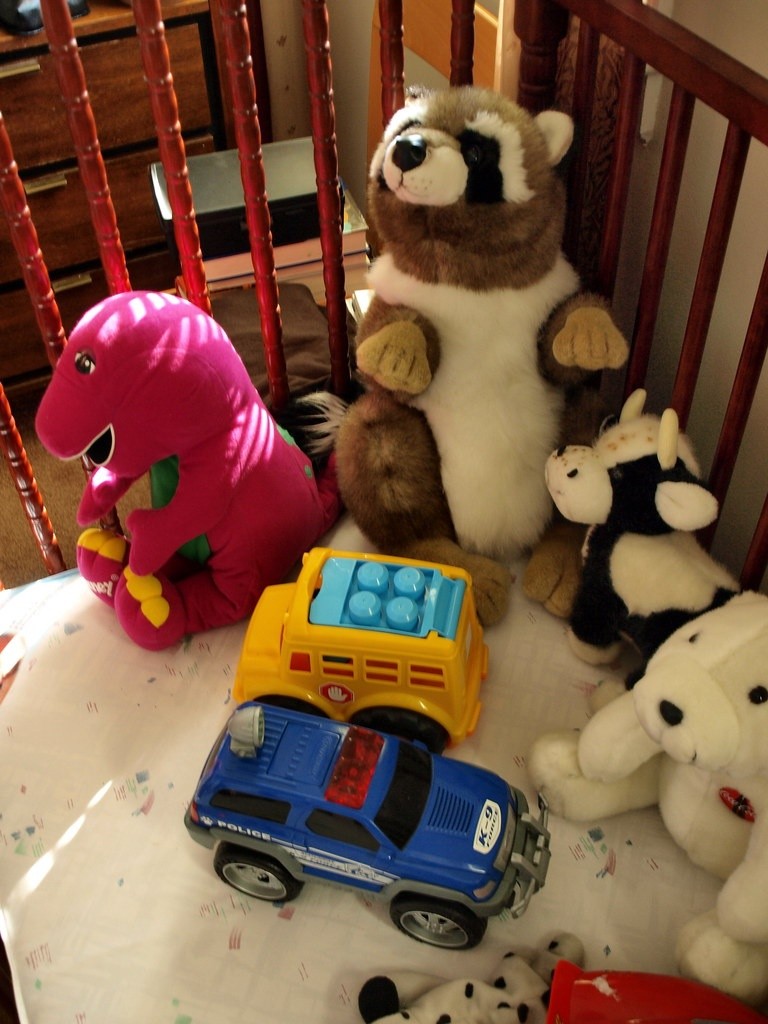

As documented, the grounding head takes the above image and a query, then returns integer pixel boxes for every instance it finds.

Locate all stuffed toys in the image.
[298,86,628,624]
[357,932,583,1023]
[37,290,340,647]
[544,388,741,717]
[529,589,768,1002]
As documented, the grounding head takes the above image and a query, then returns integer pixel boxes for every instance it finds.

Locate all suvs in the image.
[230,545,490,758]
[185,701,552,950]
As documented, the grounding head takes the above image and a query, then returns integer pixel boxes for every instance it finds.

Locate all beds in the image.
[0,0,768,1024]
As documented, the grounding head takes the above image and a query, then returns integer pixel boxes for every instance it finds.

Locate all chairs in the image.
[365,0,498,266]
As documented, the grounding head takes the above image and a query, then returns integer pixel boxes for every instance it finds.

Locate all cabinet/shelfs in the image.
[0,0,235,404]
